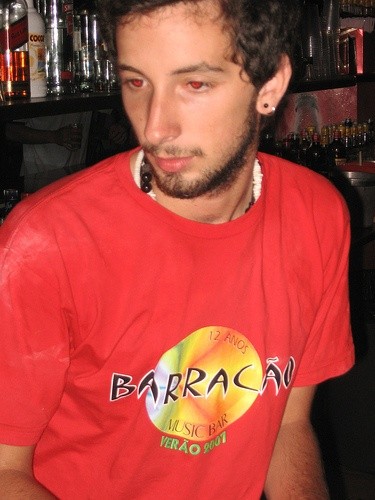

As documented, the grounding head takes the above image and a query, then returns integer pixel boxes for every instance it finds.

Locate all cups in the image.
[302,0,339,82]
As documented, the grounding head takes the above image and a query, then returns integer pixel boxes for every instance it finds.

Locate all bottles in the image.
[339,0,375,18]
[264,118,373,169]
[0,0,117,99]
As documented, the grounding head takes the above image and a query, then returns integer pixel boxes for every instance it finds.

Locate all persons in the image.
[0,0,355,500]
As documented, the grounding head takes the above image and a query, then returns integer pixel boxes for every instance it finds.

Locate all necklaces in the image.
[134,148,263,213]
